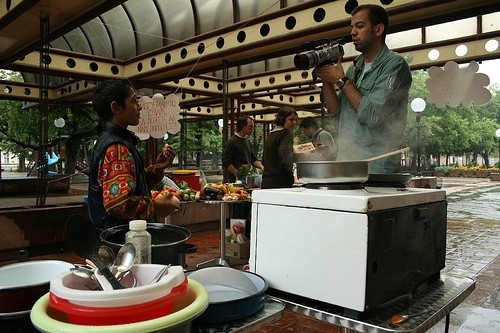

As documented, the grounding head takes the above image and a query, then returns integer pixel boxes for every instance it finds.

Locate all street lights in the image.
[410,97,426,177]
[53,117,66,172]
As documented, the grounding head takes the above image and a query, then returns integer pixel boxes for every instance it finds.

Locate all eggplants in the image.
[204,188,223,200]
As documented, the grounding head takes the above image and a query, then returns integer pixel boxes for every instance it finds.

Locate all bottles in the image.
[246,172,253,187]
[125,220,152,264]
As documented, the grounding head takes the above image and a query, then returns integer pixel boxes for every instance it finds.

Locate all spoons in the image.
[98,245,115,272]
[69,267,101,289]
[115,242,136,278]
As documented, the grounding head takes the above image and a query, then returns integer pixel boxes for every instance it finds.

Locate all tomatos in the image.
[153,187,178,199]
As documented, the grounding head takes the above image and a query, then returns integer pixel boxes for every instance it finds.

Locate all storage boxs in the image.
[165,170,202,193]
[225,241,249,259]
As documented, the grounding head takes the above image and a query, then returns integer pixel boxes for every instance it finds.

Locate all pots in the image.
[411,173,436,190]
[296,147,410,184]
[365,173,410,187]
[99,222,192,267]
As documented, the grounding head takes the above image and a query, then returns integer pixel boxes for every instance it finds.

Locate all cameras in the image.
[292,37,346,83]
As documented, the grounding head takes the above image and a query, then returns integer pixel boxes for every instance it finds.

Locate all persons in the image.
[314,4,413,174]
[261,107,297,188]
[222,116,264,184]
[85,81,180,250]
[301,116,336,161]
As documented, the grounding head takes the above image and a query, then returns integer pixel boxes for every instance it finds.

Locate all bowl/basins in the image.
[0,259,75,333]
[29,264,210,333]
[185,267,269,322]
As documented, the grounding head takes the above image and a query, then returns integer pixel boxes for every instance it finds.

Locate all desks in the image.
[189,297,286,333]
[261,267,477,333]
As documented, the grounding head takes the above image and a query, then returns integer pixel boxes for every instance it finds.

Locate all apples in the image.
[179,189,195,201]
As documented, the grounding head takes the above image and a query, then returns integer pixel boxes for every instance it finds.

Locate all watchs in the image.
[336,76,349,90]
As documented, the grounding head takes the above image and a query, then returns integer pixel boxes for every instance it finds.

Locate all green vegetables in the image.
[236,163,258,181]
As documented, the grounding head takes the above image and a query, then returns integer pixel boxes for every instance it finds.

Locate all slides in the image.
[36,159,59,175]
